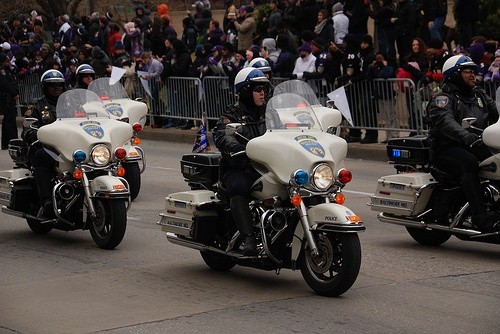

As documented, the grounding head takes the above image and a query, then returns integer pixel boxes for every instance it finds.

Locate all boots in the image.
[230,196,258,256]
[463,172,495,229]
[39,167,56,219]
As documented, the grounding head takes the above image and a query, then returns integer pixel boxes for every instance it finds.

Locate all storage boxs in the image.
[8,139,27,163]
[157,190,220,238]
[370,172,437,215]
[0,169,34,206]
[180,152,221,183]
[386,134,431,166]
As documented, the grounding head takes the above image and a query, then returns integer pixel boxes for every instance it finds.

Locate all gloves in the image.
[26,129,44,150]
[230,144,252,166]
[463,132,482,148]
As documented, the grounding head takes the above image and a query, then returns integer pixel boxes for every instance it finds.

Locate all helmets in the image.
[75,64,95,79]
[234,66,270,93]
[40,69,65,83]
[442,53,478,84]
[249,57,274,79]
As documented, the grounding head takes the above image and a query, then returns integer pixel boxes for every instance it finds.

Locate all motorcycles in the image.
[366,86,500,247]
[8,77,148,202]
[180,80,342,190]
[0,88,133,249]
[157,93,366,296]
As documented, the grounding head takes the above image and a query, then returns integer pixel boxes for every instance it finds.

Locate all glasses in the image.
[45,82,62,88]
[247,83,266,93]
[460,67,476,74]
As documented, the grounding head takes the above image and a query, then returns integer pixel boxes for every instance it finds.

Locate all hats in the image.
[0,0,500,66]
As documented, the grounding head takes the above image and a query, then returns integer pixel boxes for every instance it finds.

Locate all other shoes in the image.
[381,140,389,144]
[180,123,194,130]
[162,122,178,128]
[359,136,378,144]
[346,135,362,143]
[144,118,150,128]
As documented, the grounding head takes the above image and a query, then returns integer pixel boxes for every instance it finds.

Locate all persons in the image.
[21,70,79,218]
[0,0,500,150]
[212,67,280,255]
[426,55,500,233]
